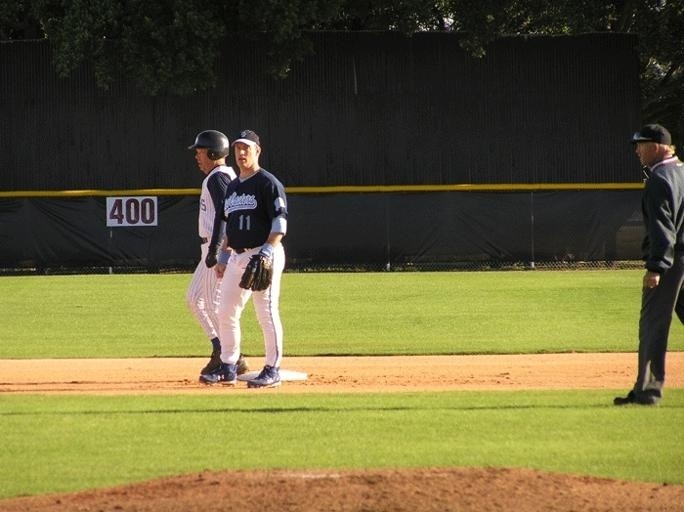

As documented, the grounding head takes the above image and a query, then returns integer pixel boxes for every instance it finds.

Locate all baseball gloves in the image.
[239,255,273,291]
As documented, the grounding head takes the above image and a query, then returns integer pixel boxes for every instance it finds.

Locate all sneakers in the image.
[200,351,247,385]
[248,365,281,389]
[611,387,658,406]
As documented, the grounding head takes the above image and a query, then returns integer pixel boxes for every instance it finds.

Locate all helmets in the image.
[188,128,229,160]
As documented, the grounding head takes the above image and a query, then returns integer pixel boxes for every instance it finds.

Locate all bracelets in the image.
[217,250,230,265]
[259,243,273,257]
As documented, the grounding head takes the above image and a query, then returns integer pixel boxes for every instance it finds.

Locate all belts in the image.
[236,248,252,255]
[199,238,208,244]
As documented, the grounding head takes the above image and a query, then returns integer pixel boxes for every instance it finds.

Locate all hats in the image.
[231,128,260,149]
[631,125,672,146]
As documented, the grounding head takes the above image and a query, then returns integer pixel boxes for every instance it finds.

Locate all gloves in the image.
[204,247,217,268]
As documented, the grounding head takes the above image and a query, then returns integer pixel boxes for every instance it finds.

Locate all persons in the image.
[614,124,684,405]
[187,131,248,378]
[199,131,289,388]
